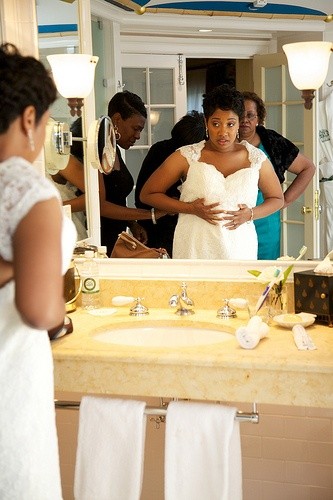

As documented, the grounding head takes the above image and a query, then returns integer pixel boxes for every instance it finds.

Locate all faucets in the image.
[169,281,195,316]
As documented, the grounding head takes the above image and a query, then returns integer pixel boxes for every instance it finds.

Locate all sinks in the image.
[89,319,239,348]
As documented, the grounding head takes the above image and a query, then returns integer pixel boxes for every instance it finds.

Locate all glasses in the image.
[241,113,258,121]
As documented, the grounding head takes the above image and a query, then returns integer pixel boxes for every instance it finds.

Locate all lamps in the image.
[46,52,99,116]
[282,41,333,110]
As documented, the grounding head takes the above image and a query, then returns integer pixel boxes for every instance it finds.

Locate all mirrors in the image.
[32,0,333,268]
[43,119,86,176]
[95,115,116,175]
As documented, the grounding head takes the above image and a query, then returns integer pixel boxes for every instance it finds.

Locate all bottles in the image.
[80,250,101,310]
[97,246,109,259]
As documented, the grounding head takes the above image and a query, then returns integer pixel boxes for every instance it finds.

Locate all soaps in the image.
[283,313,303,325]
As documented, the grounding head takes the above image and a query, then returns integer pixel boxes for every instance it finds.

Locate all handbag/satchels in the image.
[111,231,169,259]
[62,258,82,312]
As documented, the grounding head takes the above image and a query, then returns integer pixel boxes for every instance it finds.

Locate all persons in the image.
[0,42,78,500]
[50,83,316,261]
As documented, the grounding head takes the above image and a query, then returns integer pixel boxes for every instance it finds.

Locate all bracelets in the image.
[151,208,158,225]
[247,208,254,225]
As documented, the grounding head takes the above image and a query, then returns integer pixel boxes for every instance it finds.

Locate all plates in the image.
[274,313,316,328]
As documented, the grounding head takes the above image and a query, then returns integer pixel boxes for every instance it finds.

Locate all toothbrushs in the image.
[279,245,309,288]
[253,267,281,317]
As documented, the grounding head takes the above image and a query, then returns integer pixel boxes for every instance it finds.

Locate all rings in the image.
[232,220,237,226]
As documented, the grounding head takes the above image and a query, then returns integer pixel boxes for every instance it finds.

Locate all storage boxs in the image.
[292,268,333,326]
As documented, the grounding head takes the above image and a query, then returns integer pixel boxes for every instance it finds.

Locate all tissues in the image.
[292,250,333,326]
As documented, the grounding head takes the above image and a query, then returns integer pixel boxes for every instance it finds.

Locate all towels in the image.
[236,316,270,349]
[73,395,148,500]
[163,400,244,499]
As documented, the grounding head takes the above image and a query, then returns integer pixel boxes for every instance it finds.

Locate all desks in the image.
[51,242,333,408]
